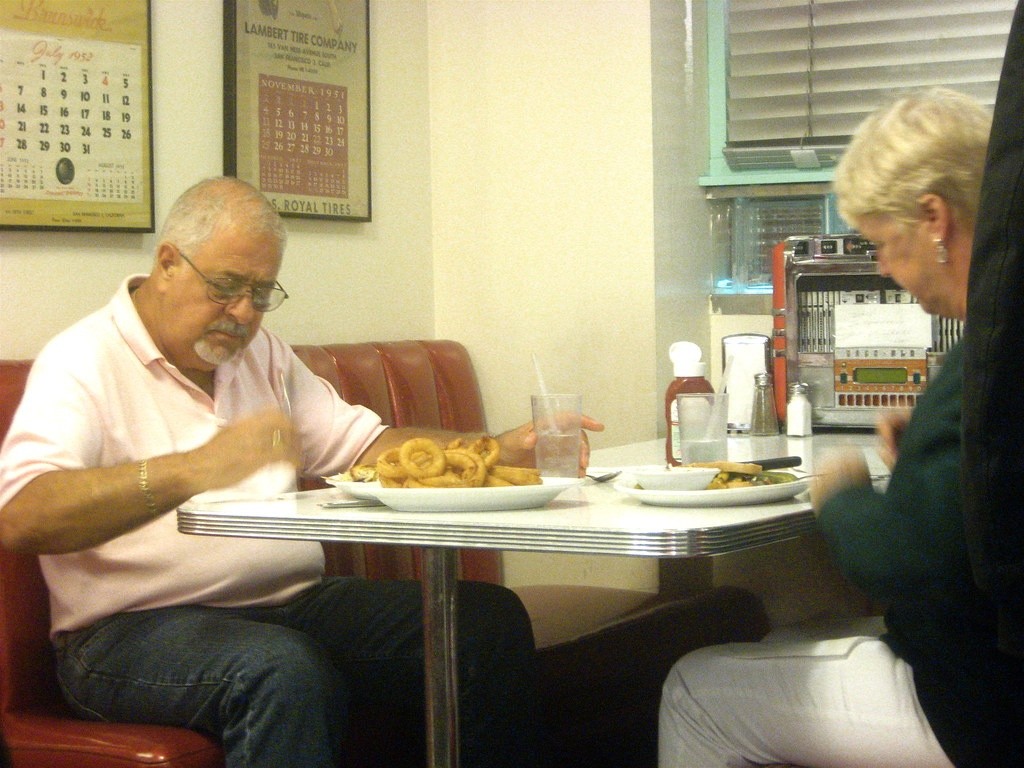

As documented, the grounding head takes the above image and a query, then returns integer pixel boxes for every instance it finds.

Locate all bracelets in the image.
[140,457,158,518]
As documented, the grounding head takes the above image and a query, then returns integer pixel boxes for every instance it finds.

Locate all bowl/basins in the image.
[627,469,720,491]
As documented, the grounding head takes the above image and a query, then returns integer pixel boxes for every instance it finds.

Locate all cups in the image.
[676,393,729,464]
[530,394,583,486]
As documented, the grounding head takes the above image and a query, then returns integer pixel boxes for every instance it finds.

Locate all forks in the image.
[584,471,622,482]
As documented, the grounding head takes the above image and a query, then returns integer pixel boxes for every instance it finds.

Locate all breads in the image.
[349,463,379,482]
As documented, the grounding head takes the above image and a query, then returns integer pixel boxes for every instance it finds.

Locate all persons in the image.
[0,176,605,768]
[961,3,1024,768]
[659,91,992,768]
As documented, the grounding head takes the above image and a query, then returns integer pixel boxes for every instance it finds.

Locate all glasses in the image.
[172,245,289,312]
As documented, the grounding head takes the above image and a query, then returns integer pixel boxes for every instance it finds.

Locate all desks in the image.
[176,428,893,768]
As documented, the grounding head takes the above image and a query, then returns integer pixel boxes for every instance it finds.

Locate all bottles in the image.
[750,373,779,437]
[787,383,812,437]
[666,362,715,467]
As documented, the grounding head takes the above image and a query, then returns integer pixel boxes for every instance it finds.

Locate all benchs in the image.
[0,340,655,768]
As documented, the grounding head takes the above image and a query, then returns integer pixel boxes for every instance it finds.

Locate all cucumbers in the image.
[754,471,797,484]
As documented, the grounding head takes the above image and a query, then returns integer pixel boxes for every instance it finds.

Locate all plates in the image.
[371,476,581,512]
[326,473,375,499]
[612,478,810,507]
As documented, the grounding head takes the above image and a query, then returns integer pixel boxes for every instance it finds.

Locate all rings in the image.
[272,429,281,447]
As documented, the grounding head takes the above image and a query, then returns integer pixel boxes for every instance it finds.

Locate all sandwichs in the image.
[687,460,763,490]
